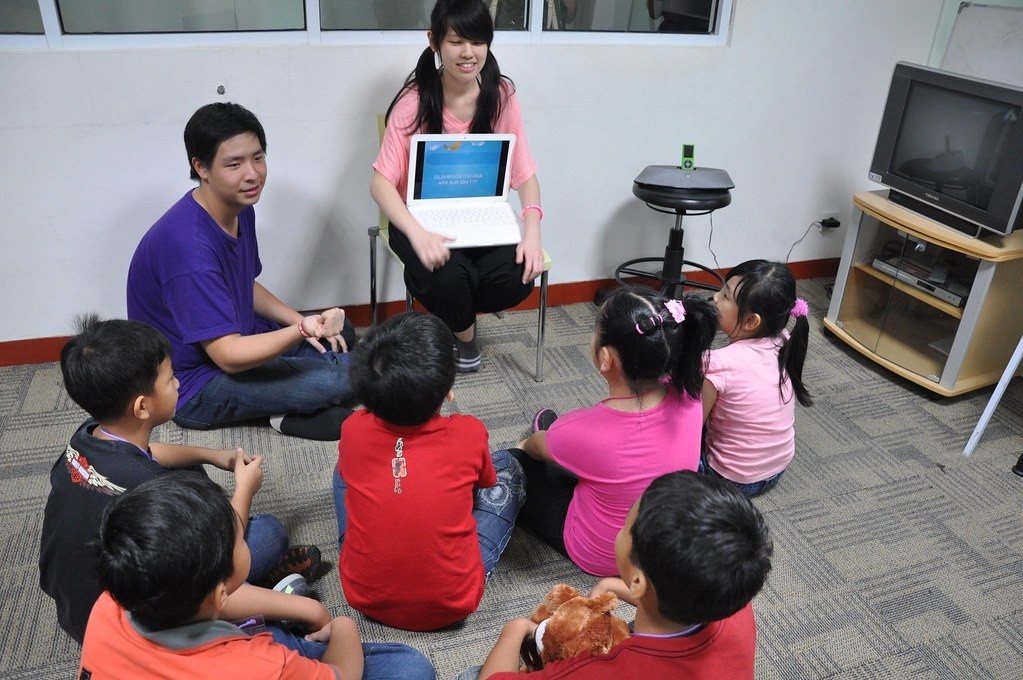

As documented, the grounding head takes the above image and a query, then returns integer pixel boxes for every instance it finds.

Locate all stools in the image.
[595,165,727,305]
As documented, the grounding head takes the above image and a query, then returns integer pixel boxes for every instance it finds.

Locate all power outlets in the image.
[818,211,840,232]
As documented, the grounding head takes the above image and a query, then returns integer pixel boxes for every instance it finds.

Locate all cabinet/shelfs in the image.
[823,190,1023,397]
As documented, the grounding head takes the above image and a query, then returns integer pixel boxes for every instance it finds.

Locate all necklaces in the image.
[97,423,151,461]
[599,384,662,403]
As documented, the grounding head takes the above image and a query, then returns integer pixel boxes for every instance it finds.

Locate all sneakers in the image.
[272,544,321,595]
[533,408,558,433]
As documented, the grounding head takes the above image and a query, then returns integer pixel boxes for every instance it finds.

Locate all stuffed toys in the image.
[519,584,630,672]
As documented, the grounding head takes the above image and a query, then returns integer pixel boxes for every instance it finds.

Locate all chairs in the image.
[368,112,551,382]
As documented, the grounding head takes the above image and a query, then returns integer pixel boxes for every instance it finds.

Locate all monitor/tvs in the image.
[869,62,1023,241]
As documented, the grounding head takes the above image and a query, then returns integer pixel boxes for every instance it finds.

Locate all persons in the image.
[509,284,719,579]
[370,0,546,369]
[332,311,526,632]
[38,313,439,680]
[457,470,774,680]
[696,260,814,498]
[127,101,364,441]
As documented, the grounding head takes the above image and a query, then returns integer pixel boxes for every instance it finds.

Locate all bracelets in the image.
[520,204,543,223]
[298,319,312,338]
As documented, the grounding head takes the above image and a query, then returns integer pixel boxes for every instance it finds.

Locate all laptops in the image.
[407,135,526,248]
